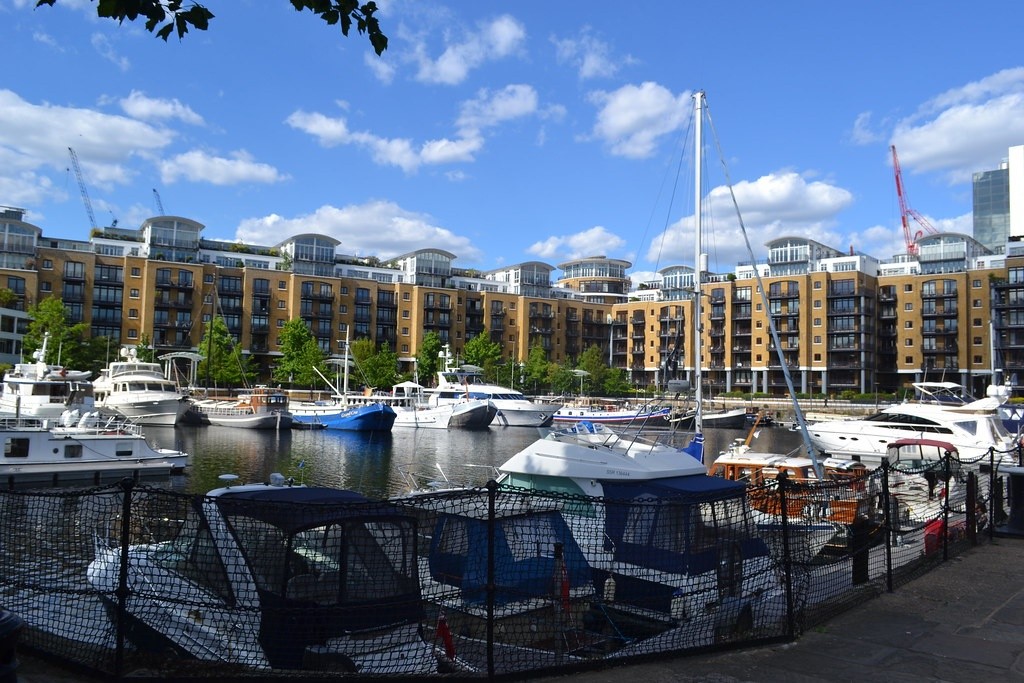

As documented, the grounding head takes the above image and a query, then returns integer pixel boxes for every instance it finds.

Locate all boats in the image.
[184,351,294,431]
[537,391,676,428]
[643,88,1024,572]
[286,476,715,672]
[90,335,194,428]
[265,324,397,433]
[1,331,190,484]
[333,325,499,431]
[425,340,563,428]
[464,420,780,631]
[85,459,441,677]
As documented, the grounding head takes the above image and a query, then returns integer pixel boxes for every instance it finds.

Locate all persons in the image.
[823,398,828,408]
